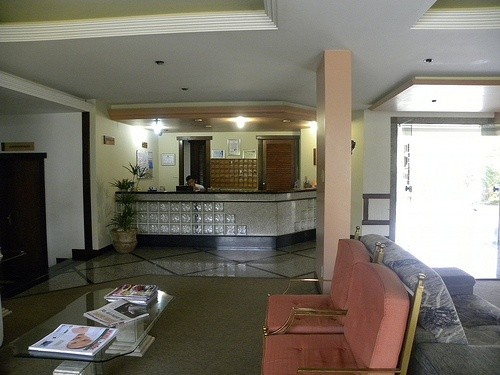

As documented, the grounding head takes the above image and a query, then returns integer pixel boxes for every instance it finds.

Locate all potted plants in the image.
[105,162,149,254]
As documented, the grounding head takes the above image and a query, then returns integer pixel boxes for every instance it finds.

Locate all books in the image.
[29,284,157,356]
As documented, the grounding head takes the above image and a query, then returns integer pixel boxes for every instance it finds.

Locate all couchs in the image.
[358,234,500,375]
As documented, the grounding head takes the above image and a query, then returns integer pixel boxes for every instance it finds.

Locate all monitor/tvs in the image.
[176,186,193,192]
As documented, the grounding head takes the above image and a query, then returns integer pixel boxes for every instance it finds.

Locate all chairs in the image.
[262,226,425,375]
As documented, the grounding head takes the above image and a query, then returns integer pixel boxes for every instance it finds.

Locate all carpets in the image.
[0,274,320,375]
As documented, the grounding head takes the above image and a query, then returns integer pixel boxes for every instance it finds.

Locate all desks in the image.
[6,287,173,375]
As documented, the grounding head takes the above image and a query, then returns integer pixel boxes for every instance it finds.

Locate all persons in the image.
[185,175,205,192]
[67,326,92,349]
